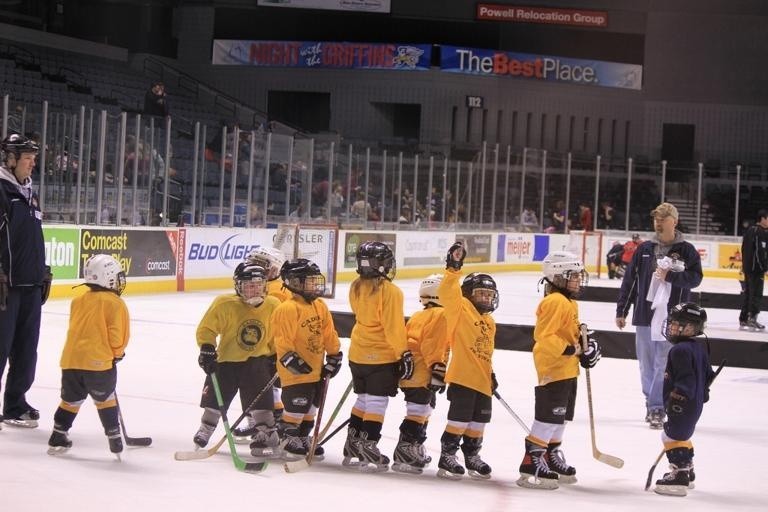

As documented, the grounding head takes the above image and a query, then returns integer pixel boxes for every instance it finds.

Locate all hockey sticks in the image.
[312,379,353,443]
[285,375,331,474]
[175,372,279,461]
[211,373,267,473]
[581,324,623,468]
[114,390,151,446]
[644,358,728,489]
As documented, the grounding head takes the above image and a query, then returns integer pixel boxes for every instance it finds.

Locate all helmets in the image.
[354,241,397,282]
[462,272,500,313]
[661,301,707,344]
[233,262,269,305]
[244,245,286,280]
[2,132,40,160]
[418,272,444,308]
[535,250,589,297]
[83,253,127,296]
[280,257,325,302]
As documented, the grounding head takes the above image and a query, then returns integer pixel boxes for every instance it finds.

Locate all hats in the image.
[650,202,679,218]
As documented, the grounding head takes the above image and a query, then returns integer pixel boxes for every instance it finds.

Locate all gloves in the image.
[445,241,467,270]
[279,350,312,375]
[398,350,414,379]
[322,351,343,379]
[580,338,602,368]
[198,344,218,375]
[427,362,447,394]
[491,373,499,394]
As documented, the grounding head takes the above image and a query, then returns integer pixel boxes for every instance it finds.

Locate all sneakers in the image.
[544,440,576,477]
[4,405,40,421]
[192,421,216,448]
[645,406,650,422]
[459,441,492,475]
[48,419,73,448]
[357,427,390,464]
[655,441,691,487]
[249,423,279,449]
[275,418,307,460]
[416,430,432,463]
[662,441,695,482]
[739,316,758,329]
[749,312,765,329]
[343,423,360,458]
[393,428,426,468]
[233,422,255,438]
[438,439,465,475]
[105,423,123,453]
[650,410,666,429]
[519,436,560,480]
[299,420,325,456]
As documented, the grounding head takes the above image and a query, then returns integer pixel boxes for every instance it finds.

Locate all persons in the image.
[738,209,768,332]
[606,239,625,280]
[601,201,616,229]
[577,201,592,231]
[192,260,281,459]
[544,200,565,233]
[391,272,451,475]
[519,207,540,234]
[515,250,602,490]
[0,132,53,429]
[341,240,415,473]
[210,120,471,230]
[615,203,704,429]
[232,245,291,443]
[621,232,643,263]
[436,241,500,480]
[270,257,343,462]
[47,253,131,462]
[142,82,170,128]
[653,301,716,497]
[90,134,165,187]
